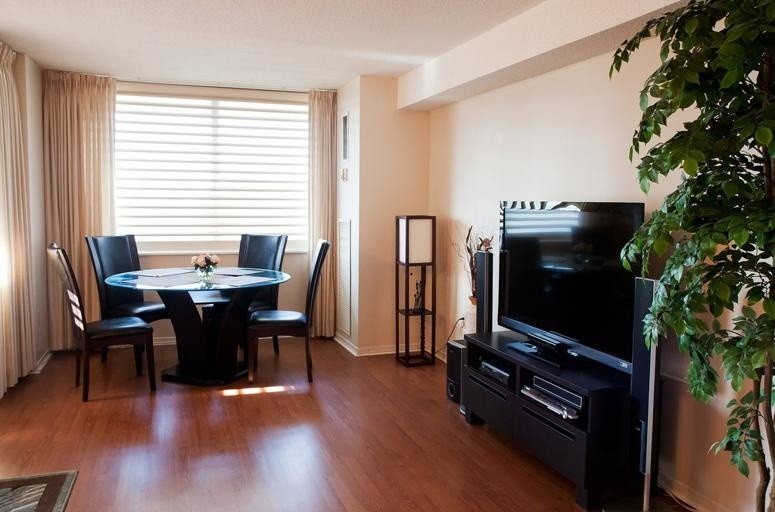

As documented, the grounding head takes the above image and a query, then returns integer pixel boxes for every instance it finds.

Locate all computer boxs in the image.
[447,340,469,404]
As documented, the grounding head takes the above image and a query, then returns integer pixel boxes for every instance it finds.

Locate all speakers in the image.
[626,276,664,512]
[474,250,494,340]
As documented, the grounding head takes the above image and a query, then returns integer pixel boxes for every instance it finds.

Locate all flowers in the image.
[448,223,493,295]
[191,253,220,277]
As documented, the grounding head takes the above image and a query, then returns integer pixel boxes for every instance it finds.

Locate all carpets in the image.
[0,470,80,511]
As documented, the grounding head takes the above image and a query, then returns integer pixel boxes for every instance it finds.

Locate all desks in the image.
[104,268,288,388]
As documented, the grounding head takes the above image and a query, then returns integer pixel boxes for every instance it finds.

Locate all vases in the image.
[200,269,214,281]
[461,294,477,336]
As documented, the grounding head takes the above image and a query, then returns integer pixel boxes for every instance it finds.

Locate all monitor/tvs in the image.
[496,201,645,376]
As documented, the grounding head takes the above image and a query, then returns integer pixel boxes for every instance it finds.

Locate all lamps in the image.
[395,214,436,366]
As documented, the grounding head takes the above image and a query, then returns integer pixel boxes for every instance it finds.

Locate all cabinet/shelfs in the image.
[463,330,631,511]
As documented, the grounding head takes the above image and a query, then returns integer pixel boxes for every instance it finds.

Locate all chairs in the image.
[46,233,171,402]
[234,230,329,385]
